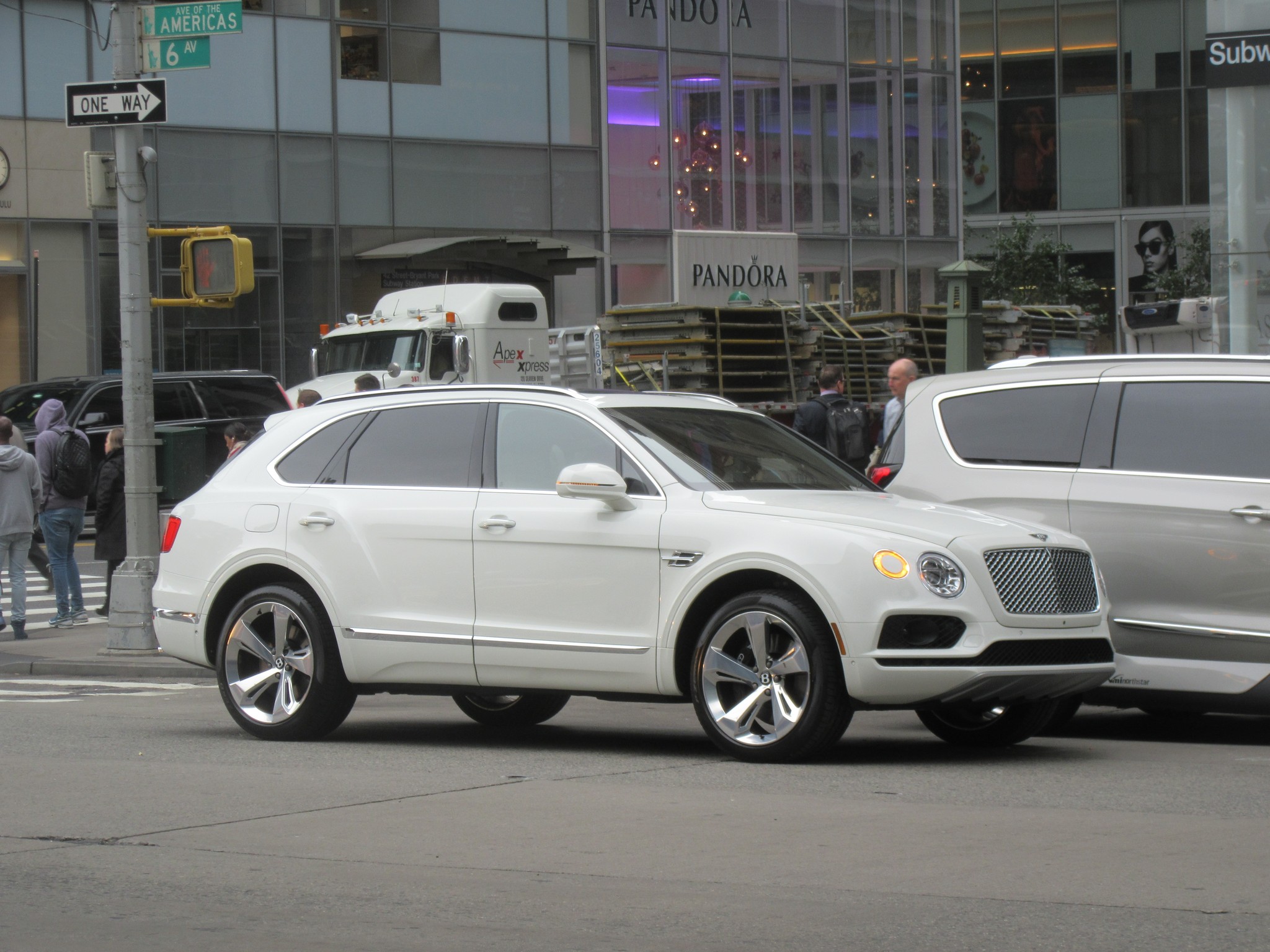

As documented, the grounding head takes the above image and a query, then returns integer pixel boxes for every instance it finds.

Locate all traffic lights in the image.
[185,235,255,299]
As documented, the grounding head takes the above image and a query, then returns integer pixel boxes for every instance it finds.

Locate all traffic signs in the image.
[63,78,167,128]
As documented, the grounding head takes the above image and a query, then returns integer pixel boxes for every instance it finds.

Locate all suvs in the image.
[0,369,295,543]
[152,383,1116,767]
[863,354,1269,738]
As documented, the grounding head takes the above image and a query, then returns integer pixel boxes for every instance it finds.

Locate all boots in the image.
[10,619,28,640]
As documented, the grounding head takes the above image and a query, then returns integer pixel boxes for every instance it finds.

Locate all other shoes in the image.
[96,607,108,617]
[47,575,54,592]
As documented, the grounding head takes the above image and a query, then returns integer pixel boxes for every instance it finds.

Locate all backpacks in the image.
[47,426,92,499]
[810,395,871,470]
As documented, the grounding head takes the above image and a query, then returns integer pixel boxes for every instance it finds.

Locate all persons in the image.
[1128,220,1183,305]
[0,416,44,639]
[34,399,88,629]
[876,358,917,465]
[8,425,55,593]
[792,365,870,479]
[295,388,323,409]
[864,418,882,476]
[95,428,127,615]
[354,373,381,393]
[224,422,255,461]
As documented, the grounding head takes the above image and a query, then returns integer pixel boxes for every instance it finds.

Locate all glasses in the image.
[1134,241,1168,256]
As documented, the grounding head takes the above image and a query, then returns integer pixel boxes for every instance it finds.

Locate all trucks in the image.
[284,280,606,410]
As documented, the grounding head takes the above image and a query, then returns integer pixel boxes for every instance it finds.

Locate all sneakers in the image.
[70,607,89,624]
[49,613,73,629]
